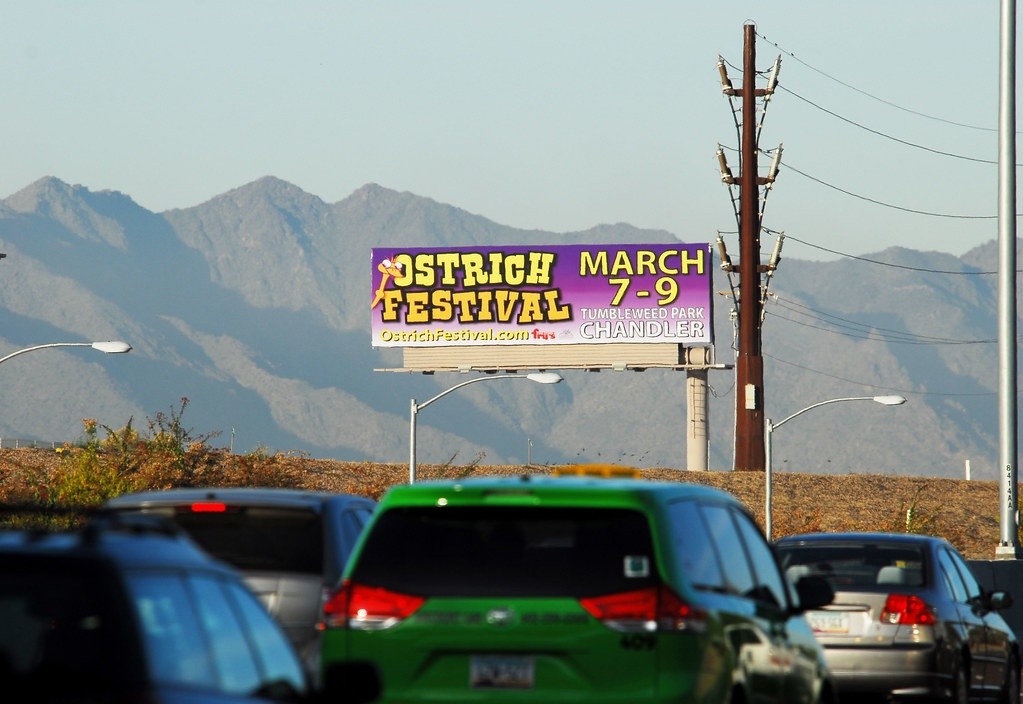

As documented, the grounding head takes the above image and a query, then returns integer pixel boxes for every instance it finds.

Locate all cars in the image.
[765,530,1023,703]
[89,490,378,669]
[307,473,841,704]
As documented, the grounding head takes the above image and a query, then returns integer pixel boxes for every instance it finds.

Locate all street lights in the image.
[403,372,566,484]
[765,394,906,540]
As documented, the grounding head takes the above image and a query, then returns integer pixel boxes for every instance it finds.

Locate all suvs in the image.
[0,500,312,704]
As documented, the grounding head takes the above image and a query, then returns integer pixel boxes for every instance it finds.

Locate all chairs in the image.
[877,566,905,584]
